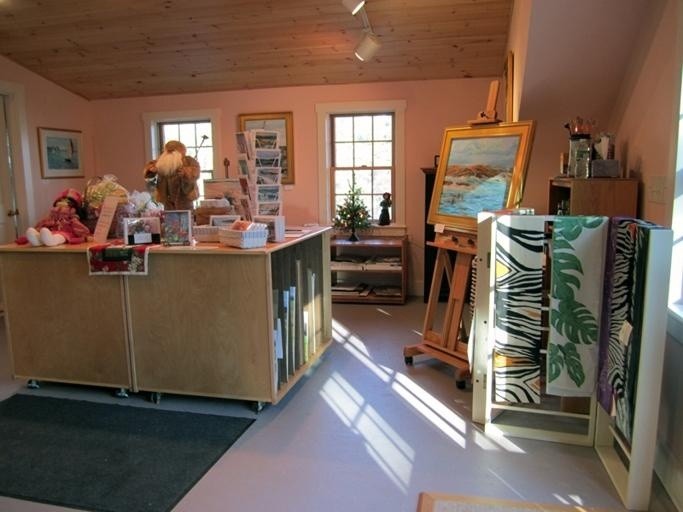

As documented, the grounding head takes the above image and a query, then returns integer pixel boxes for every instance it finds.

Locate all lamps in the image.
[351,1,379,64]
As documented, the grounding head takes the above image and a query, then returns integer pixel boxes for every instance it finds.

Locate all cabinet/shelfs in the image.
[329,234,409,306]
[542,174,640,416]
[0,230,334,415]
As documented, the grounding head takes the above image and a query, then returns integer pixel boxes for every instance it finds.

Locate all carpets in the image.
[0,394,257,512]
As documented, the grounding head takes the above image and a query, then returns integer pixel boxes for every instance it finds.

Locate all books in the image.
[229,129,286,244]
[163,209,192,245]
[330,255,402,297]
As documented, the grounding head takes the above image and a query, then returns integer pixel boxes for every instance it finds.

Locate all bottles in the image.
[574,137,591,180]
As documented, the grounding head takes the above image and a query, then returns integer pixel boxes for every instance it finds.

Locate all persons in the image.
[378,193,393,226]
[141,140,203,211]
[24,187,93,247]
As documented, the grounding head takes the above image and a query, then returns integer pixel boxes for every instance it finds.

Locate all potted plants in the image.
[331,186,373,242]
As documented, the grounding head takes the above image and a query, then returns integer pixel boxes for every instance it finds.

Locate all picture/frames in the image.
[37,126,85,179]
[426,121,537,232]
[238,111,295,185]
